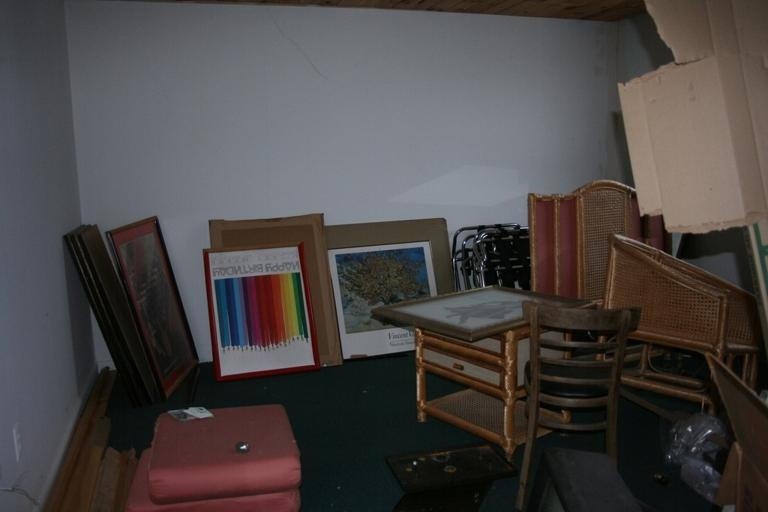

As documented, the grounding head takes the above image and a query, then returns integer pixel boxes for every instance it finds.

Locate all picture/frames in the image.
[385,442,520,495]
[371,284,598,344]
[61,215,202,408]
[202,241,321,383]
[327,239,439,363]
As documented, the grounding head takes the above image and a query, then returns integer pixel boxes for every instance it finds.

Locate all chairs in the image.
[593,232,763,427]
[515,299,642,511]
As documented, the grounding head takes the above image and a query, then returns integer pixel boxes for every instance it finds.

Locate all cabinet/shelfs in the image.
[415,325,573,464]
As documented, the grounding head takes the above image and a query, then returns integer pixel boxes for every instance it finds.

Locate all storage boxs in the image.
[705,349,767,512]
